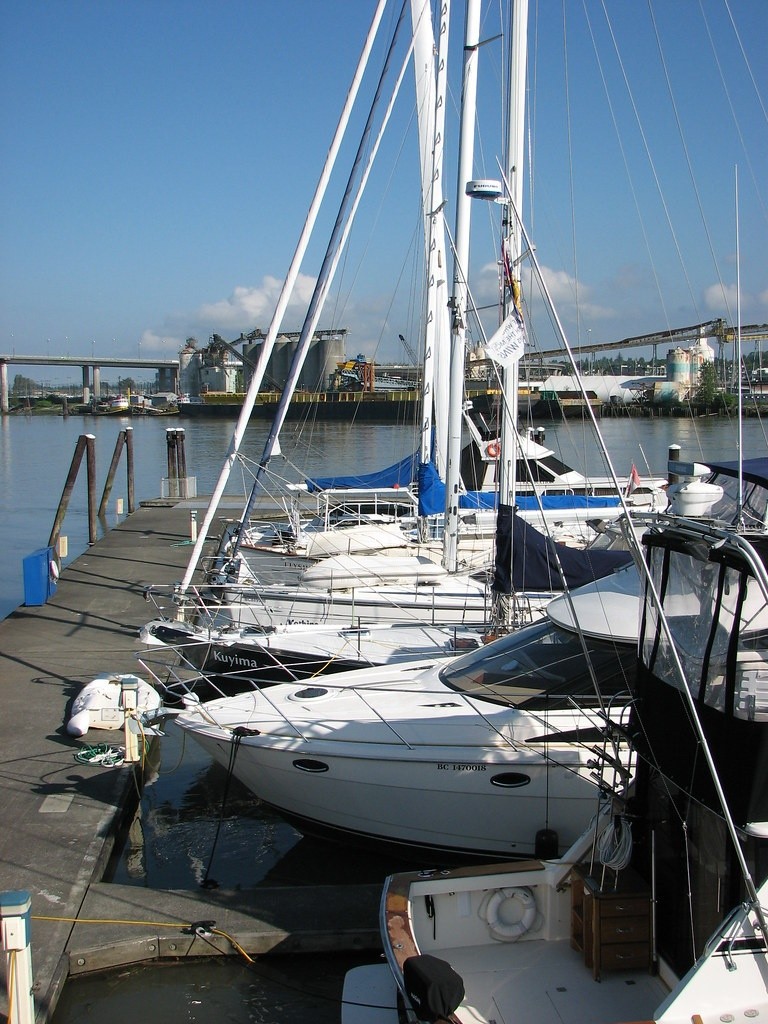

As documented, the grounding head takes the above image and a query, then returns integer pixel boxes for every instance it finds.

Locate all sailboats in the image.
[132,0,767,1024]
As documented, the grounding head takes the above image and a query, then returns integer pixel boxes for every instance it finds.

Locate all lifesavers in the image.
[487,443,501,457]
[484,887,537,937]
